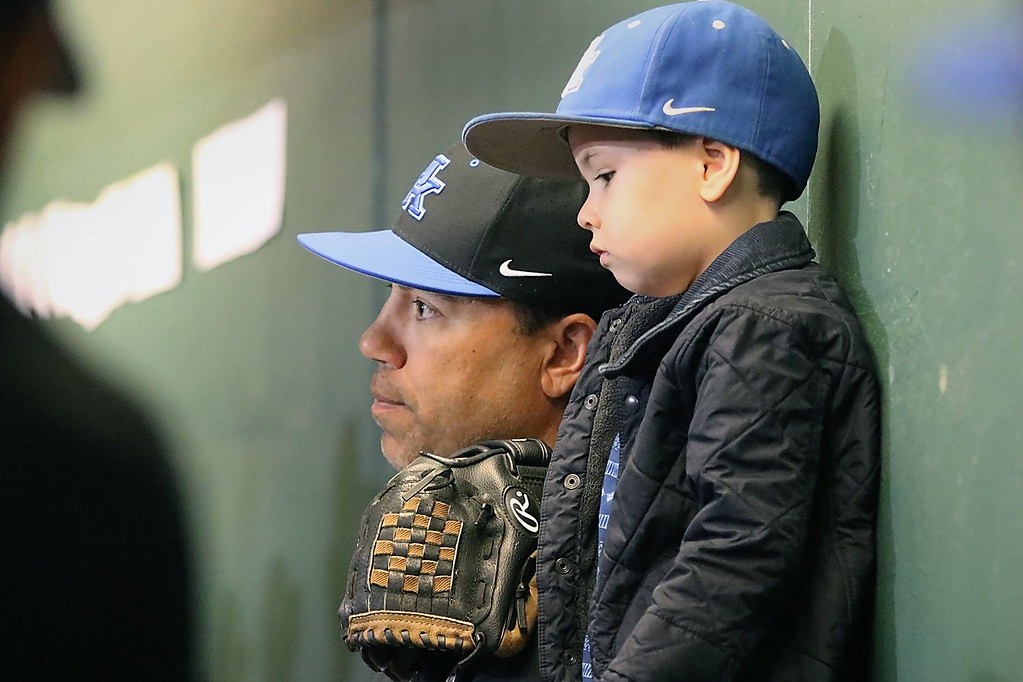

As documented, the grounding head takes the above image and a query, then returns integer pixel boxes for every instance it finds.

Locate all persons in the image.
[298,143,633,682]
[461,0,883,681]
[0,0,211,682]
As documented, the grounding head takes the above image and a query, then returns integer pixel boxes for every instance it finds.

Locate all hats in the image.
[461,0,820,201]
[296,141,632,319]
